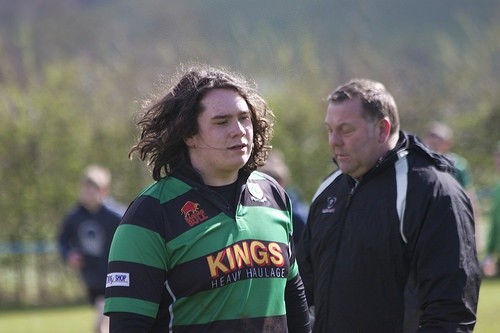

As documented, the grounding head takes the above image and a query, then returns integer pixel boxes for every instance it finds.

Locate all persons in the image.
[476,137,500,295]
[101,60,317,333]
[251,145,316,332]
[49,165,128,333]
[292,78,482,333]
[417,118,472,202]
